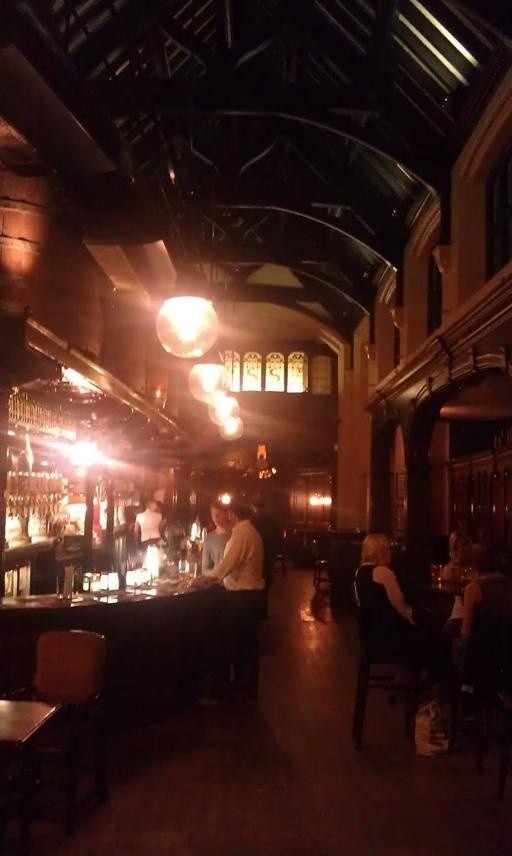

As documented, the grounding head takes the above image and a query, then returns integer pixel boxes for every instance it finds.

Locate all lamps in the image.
[153,262,247,442]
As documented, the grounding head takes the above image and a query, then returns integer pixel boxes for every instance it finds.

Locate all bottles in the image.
[5,470,67,517]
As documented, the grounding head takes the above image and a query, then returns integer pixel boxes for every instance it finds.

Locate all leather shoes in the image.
[199,696,225,709]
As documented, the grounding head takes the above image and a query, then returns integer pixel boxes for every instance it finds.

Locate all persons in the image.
[351,520,511,739]
[131,495,289,703]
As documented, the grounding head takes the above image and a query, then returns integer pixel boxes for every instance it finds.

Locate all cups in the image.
[430,563,443,584]
[56,575,78,600]
[458,567,472,590]
[184,562,197,580]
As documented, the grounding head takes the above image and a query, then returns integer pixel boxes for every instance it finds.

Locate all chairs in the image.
[312,539,332,588]
[18,628,110,855]
[269,529,289,577]
[350,604,416,751]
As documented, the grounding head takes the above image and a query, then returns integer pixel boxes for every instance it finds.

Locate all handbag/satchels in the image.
[414,699,453,756]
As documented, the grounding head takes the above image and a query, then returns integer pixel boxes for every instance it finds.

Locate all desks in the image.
[0,699,64,855]
